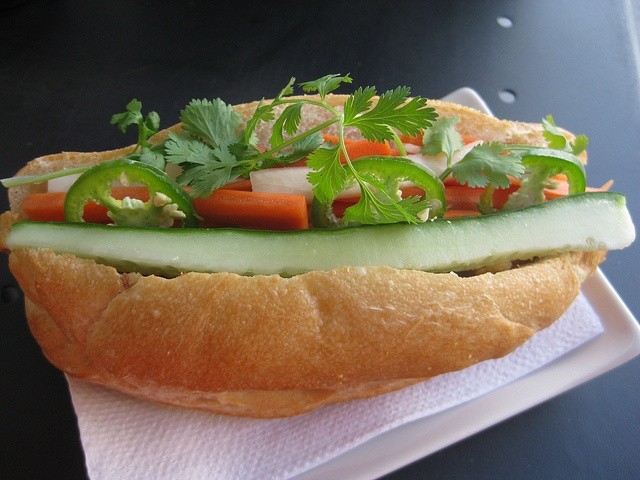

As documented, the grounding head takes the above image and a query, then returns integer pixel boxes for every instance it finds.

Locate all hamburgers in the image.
[1,72,638,419]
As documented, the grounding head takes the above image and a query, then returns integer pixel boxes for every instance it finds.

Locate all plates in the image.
[66,87,636,480]
